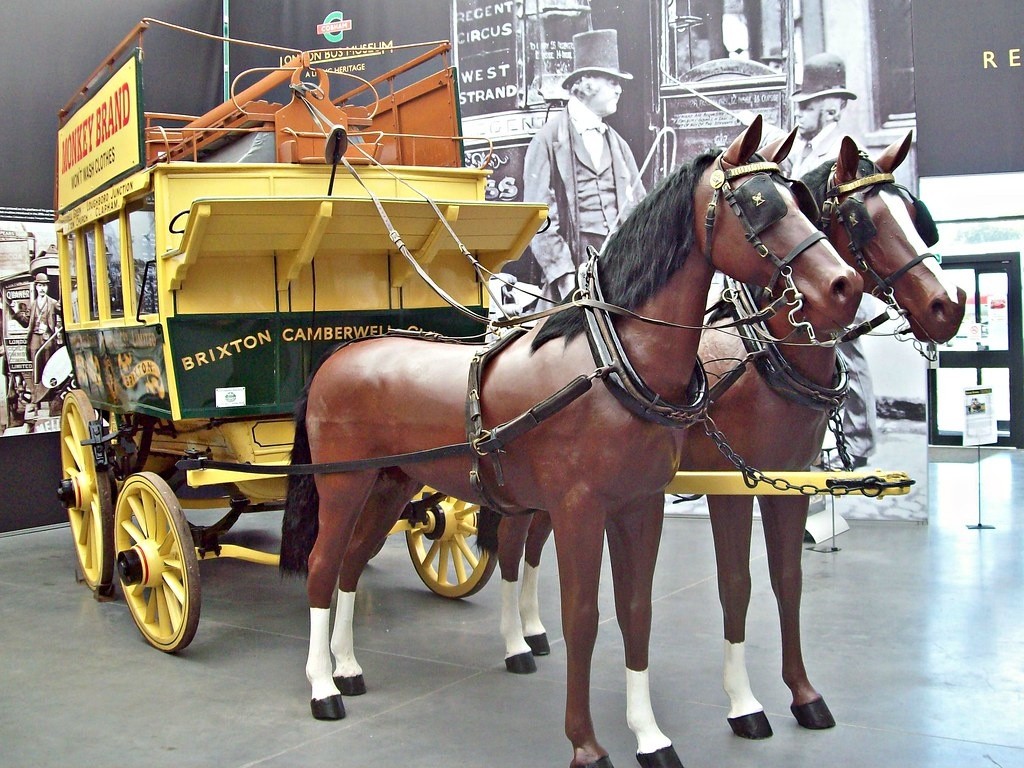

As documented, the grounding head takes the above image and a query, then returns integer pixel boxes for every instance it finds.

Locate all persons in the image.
[521,29,649,324]
[7,273,61,411]
[788,54,878,470]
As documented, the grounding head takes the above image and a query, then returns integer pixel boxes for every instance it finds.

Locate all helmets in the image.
[790,54,857,104]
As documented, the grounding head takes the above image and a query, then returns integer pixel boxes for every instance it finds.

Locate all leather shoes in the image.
[824,454,869,472]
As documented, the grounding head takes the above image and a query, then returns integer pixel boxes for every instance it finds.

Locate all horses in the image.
[278,113,865,768]
[474,130,967,739]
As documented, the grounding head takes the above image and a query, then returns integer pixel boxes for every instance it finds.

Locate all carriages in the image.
[44,16,967,768]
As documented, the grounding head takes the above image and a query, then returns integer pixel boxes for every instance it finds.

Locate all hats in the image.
[34,273,49,283]
[562,28,633,91]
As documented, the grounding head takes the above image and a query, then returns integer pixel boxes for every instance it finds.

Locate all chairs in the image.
[274,67,384,167]
